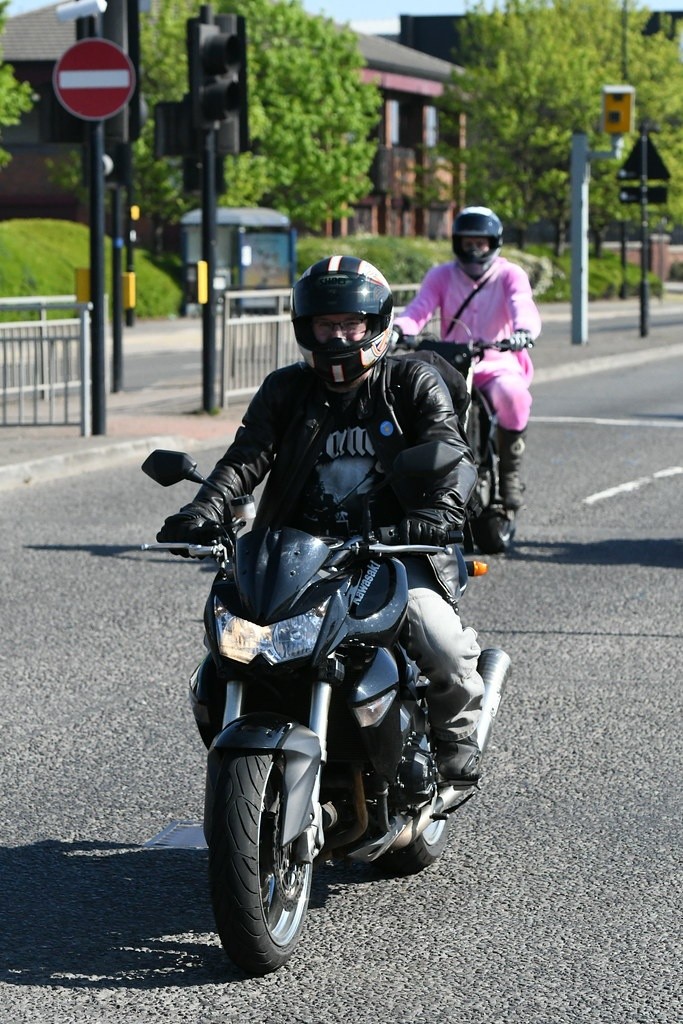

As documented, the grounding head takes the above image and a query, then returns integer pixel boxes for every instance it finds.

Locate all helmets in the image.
[290,255,394,387]
[451,206,504,278]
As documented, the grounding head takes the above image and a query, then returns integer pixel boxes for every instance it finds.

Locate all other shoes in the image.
[430,730,480,780]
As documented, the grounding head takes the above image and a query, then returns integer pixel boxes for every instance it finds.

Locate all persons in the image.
[390,205,541,510]
[154,256,484,794]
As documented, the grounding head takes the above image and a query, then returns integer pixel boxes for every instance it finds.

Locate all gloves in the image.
[508,330,527,351]
[390,325,401,350]
[399,508,456,556]
[156,510,208,559]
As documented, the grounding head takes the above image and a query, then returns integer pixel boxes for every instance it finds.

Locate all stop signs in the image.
[53,37,138,122]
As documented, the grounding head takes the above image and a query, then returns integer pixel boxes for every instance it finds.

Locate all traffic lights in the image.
[186,18,243,134]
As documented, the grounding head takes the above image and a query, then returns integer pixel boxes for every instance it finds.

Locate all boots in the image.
[496,425,527,511]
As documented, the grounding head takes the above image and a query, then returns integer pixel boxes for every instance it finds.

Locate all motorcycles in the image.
[391,327,545,552]
[137,441,519,978]
